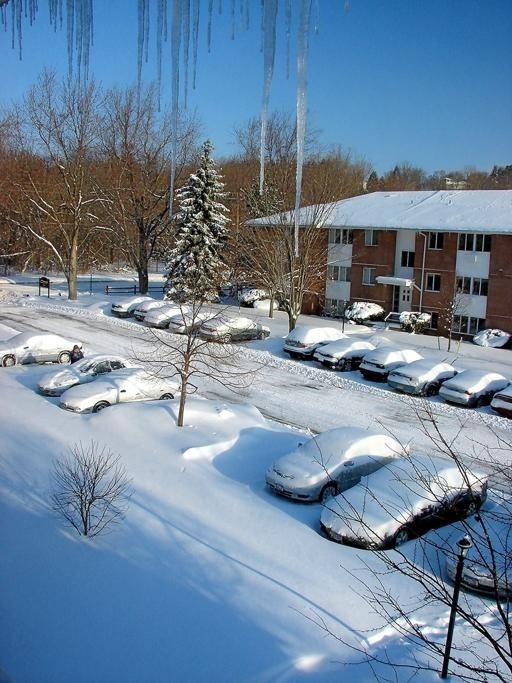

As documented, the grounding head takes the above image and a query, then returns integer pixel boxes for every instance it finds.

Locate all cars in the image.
[111,295,271,344]
[1,330,174,414]
[283,326,512,416]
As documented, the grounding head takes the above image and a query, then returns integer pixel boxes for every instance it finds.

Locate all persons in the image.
[71,345,84,365]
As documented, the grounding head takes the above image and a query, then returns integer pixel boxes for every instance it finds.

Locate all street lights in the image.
[375,233,427,312]
[441,532,474,679]
